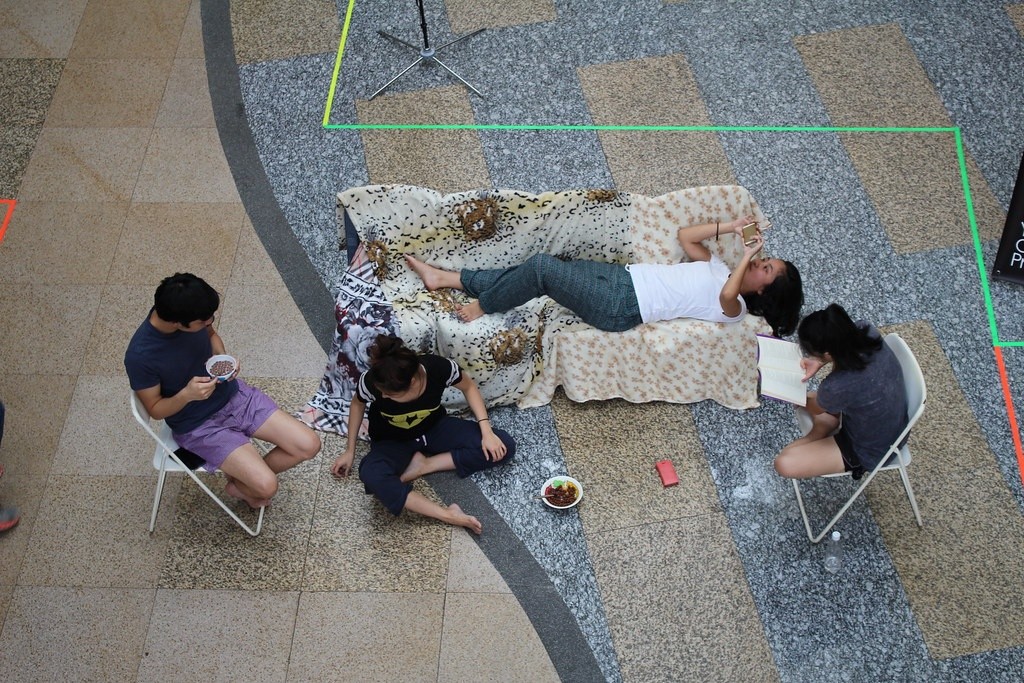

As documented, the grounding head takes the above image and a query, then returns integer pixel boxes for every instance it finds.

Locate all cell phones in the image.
[169,447,206,471]
[743,222,757,245]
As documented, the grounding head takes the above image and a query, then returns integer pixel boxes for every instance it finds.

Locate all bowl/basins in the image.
[540,476,583,509]
[206,354,237,381]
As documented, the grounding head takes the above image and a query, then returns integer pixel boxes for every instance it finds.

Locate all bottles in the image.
[825,531,843,574]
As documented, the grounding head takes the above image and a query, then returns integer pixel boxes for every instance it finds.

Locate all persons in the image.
[405,213,804,337]
[774,303,912,478]
[124,273,321,508]
[330,332,516,534]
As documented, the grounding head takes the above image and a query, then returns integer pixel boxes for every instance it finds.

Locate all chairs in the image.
[794,331,928,544]
[131,389,265,539]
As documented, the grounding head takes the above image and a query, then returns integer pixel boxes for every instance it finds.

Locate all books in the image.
[756,334,807,407]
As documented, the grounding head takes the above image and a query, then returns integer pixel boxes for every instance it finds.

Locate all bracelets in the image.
[478,418,489,422]
[716,222,719,241]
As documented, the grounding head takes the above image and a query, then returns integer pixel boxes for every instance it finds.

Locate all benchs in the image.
[296,179,771,447]
